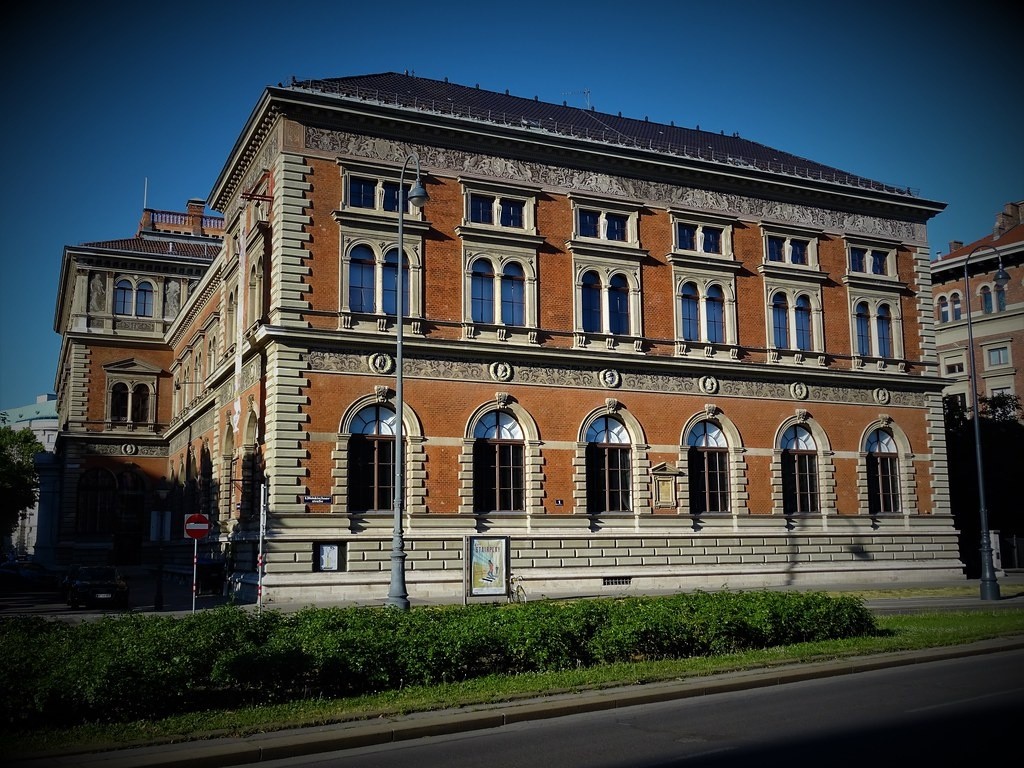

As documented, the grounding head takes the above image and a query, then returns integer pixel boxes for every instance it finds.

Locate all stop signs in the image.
[185,514,211,538]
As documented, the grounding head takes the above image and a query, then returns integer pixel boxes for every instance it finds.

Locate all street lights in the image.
[386,153,431,605]
[964,245,1012,601]
[152,475,172,611]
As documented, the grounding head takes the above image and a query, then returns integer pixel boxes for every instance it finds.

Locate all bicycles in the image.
[508,572,527,604]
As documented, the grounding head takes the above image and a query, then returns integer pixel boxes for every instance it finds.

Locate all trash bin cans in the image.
[196,557,225,595]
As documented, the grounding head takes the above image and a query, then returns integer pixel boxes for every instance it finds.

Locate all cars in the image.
[64,563,134,611]
